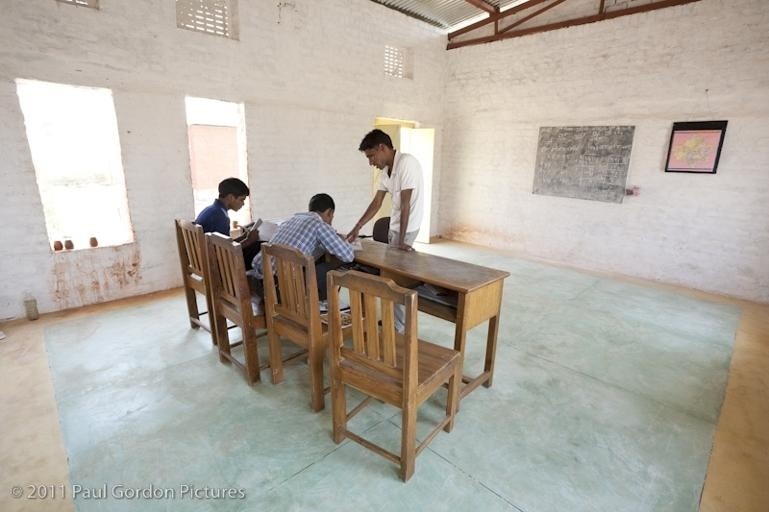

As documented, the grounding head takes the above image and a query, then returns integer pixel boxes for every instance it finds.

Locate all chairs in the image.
[175,218,463,483]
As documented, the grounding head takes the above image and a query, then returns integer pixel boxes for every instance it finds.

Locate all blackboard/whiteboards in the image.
[532,126,635,204]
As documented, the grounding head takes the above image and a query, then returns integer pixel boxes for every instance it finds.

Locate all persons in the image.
[247,190,356,313]
[196,178,263,275]
[343,129,426,335]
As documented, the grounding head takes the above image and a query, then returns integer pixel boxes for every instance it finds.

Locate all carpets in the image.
[45,243,739,512]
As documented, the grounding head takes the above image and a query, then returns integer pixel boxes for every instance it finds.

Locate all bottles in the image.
[24,290,39,320]
